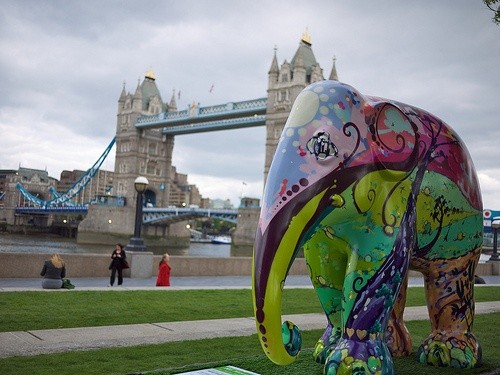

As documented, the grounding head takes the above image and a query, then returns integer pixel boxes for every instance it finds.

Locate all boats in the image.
[189,228,232,244]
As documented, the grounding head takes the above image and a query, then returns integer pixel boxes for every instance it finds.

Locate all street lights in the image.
[126,176,150,251]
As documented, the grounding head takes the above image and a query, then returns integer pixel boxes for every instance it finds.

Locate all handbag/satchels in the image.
[61,278,75,289]
[122,260,129,269]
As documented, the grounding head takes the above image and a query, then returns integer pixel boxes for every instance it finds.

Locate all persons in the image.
[155,254,171,286]
[40,254,65,289]
[108,243,129,287]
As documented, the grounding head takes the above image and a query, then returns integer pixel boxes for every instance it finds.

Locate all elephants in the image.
[252,79,483,375]
